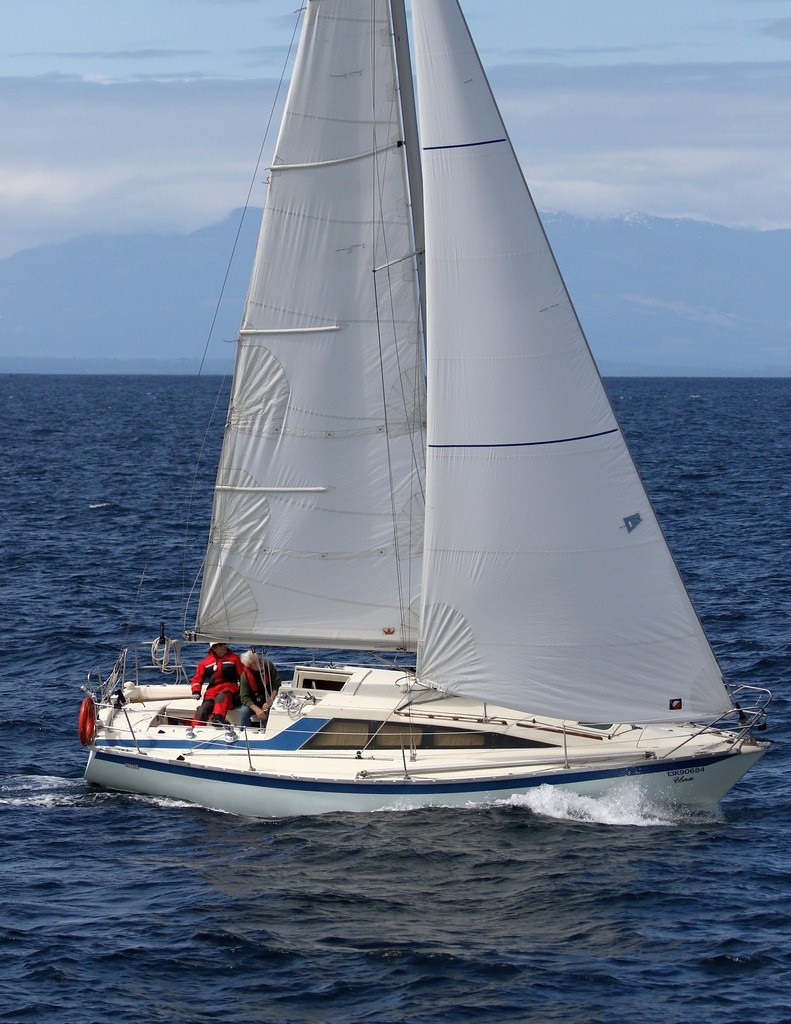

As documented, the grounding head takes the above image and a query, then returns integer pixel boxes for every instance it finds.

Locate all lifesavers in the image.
[78,697,95,747]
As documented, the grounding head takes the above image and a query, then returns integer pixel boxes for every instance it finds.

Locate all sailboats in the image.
[86,7,772,833]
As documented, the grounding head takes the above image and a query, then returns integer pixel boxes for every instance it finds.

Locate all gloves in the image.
[193,694,201,701]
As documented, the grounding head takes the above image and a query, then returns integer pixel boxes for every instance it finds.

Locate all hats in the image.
[207,641,220,650]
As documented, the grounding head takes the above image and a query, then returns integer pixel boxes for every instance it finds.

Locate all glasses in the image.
[219,643,228,646]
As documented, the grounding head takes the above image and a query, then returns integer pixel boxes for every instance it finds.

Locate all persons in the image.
[189,642,245,727]
[238,650,281,731]
